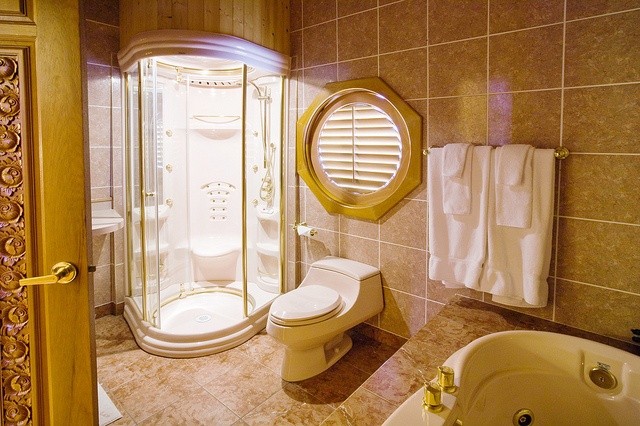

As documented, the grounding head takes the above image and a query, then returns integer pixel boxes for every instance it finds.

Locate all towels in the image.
[442,143,475,217]
[441,142,470,179]
[496,146,535,228]
[495,143,532,185]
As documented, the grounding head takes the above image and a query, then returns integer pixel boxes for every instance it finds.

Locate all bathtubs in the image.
[381,329,640,426]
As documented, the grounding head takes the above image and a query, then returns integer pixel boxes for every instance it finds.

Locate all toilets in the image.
[266,255,385,383]
